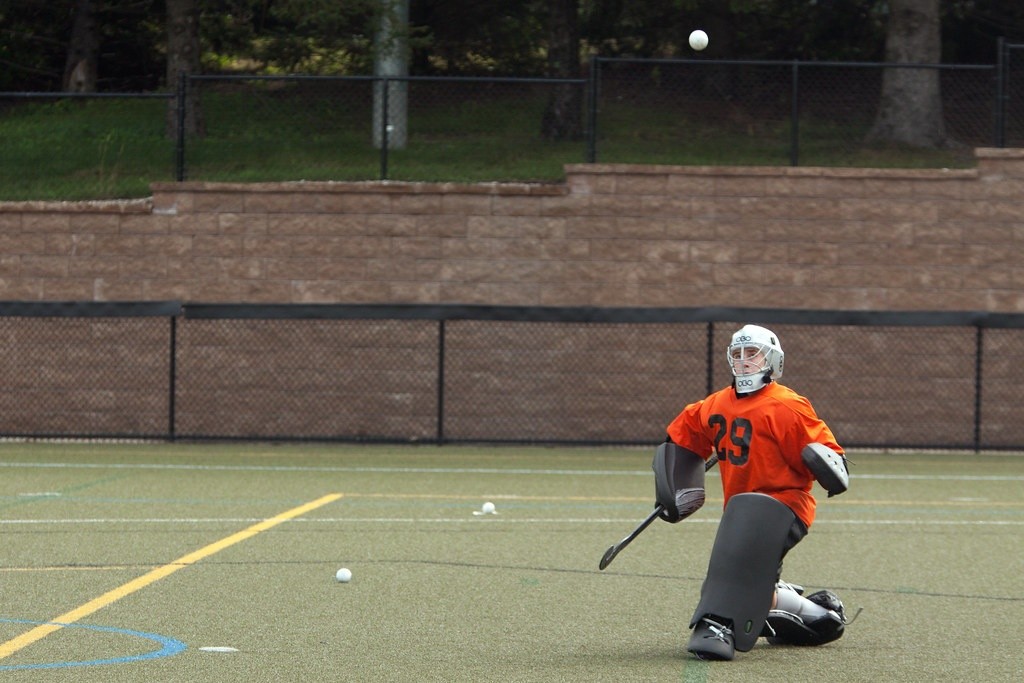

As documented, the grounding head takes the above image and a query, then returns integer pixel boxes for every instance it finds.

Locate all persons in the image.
[651,325,850,661]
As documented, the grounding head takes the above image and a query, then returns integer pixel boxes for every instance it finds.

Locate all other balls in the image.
[689,30,709,50]
[337,569,351,583]
[483,502,495,515]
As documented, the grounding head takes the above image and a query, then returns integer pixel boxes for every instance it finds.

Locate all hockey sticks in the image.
[600,453,717,570]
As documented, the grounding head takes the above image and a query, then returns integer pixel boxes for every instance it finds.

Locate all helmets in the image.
[727,324,784,393]
